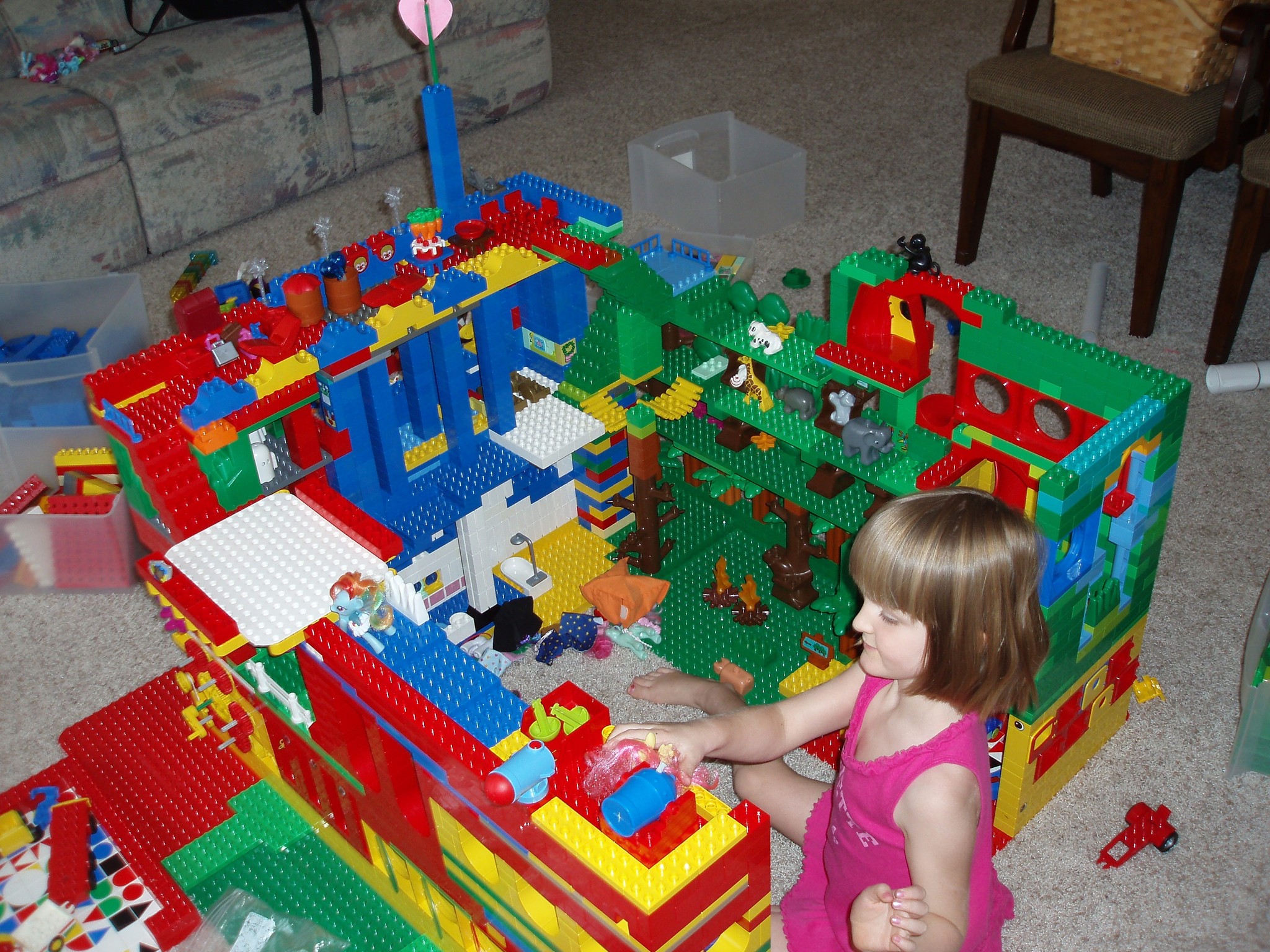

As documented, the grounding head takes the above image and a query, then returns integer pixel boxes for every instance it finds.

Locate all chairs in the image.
[954,0,1270,364]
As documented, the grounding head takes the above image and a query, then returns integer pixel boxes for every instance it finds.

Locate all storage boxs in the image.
[628,111,806,239]
[0,275,148,427]
[625,215,755,286]
[0,426,147,595]
[1223,571,1270,782]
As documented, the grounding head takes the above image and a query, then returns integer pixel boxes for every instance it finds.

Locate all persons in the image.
[600,486,1049,952]
